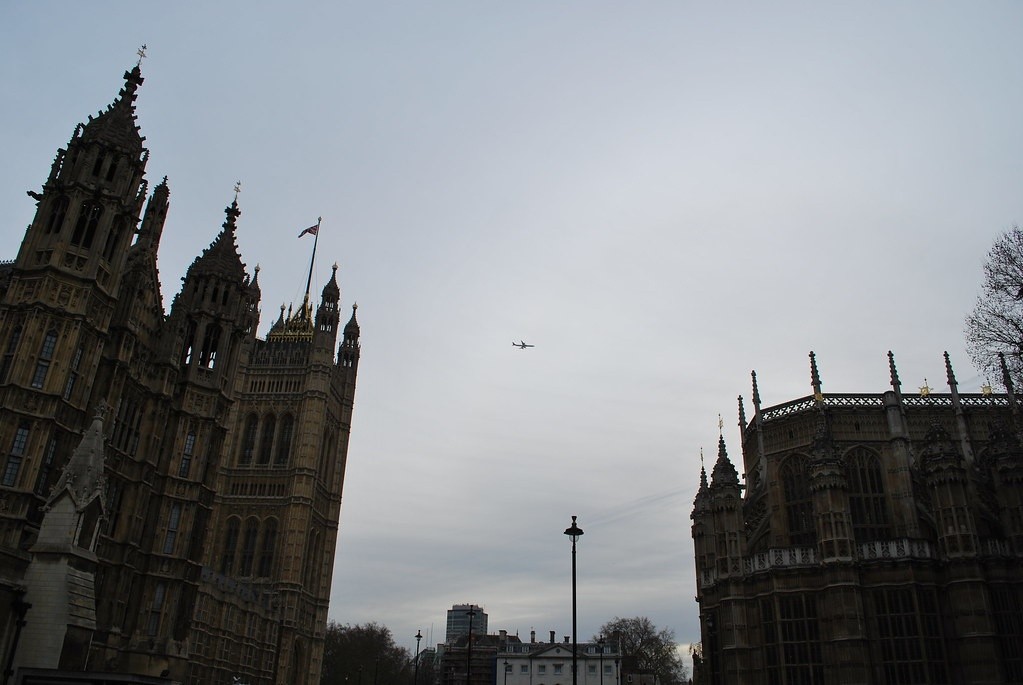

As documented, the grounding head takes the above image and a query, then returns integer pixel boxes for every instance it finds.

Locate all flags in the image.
[298,225,317,238]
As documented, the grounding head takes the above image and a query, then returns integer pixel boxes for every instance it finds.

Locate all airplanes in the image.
[511,340,536,350]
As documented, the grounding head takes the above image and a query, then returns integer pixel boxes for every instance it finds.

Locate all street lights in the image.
[375,656,379,685]
[465,604,477,685]
[503,661,509,685]
[358,665,363,685]
[705,617,717,685]
[563,514,587,685]
[413,629,423,685]
[596,635,607,685]
